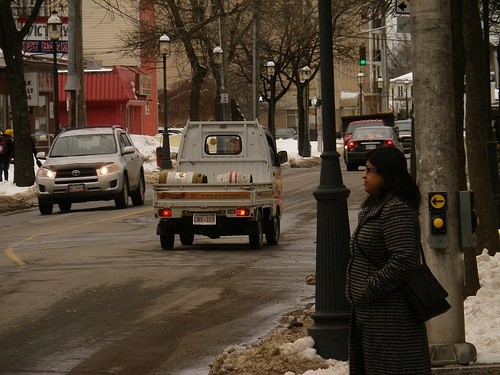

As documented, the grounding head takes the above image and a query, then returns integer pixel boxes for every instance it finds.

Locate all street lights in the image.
[357,70,365,120]
[212,44,225,122]
[159,32,171,169]
[301,64,312,158]
[389,76,396,121]
[312,96,318,142]
[403,78,410,120]
[377,76,384,119]
[265,56,277,148]
[47,5,63,138]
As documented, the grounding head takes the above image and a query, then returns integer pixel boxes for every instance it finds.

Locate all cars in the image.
[154,127,184,149]
[344,125,405,171]
[275,128,297,141]
[344,120,386,164]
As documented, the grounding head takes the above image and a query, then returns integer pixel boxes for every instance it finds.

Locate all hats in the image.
[5,129,13,134]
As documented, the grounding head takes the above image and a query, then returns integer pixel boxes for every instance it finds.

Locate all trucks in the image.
[151,118,288,250]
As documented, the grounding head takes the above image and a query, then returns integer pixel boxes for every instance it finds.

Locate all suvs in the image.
[33,124,146,215]
[394,119,412,154]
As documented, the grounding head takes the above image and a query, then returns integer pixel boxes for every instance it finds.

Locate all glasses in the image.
[365,166,377,174]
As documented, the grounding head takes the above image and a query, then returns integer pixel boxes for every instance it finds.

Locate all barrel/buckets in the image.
[158,171,207,184]
[218,171,253,185]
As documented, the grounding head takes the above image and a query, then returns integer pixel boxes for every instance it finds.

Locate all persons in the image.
[0,129,13,182]
[346,146,431,375]
[32,137,37,154]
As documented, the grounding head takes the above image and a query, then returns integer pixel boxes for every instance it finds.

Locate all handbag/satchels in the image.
[398,264,452,324]
[35,157,42,167]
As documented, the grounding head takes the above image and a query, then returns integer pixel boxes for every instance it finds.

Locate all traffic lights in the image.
[359,45,366,66]
